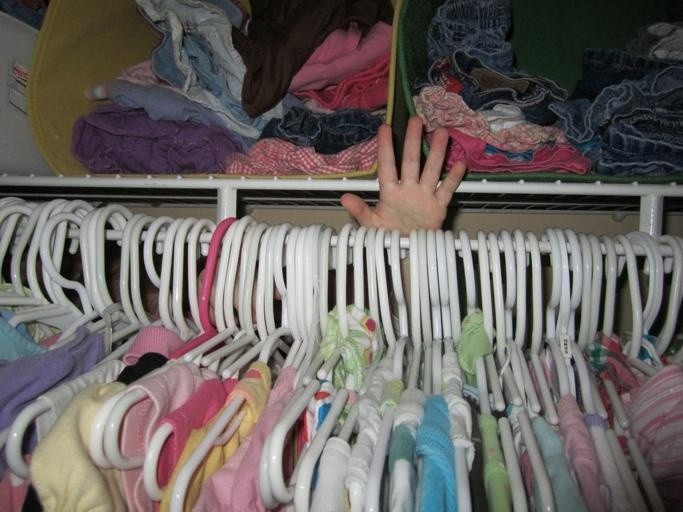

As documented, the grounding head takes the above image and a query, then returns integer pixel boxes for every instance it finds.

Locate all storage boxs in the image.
[29,2,401,181]
[396,1,682,185]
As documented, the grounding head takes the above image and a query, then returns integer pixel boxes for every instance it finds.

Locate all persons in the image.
[80,117,467,361]
